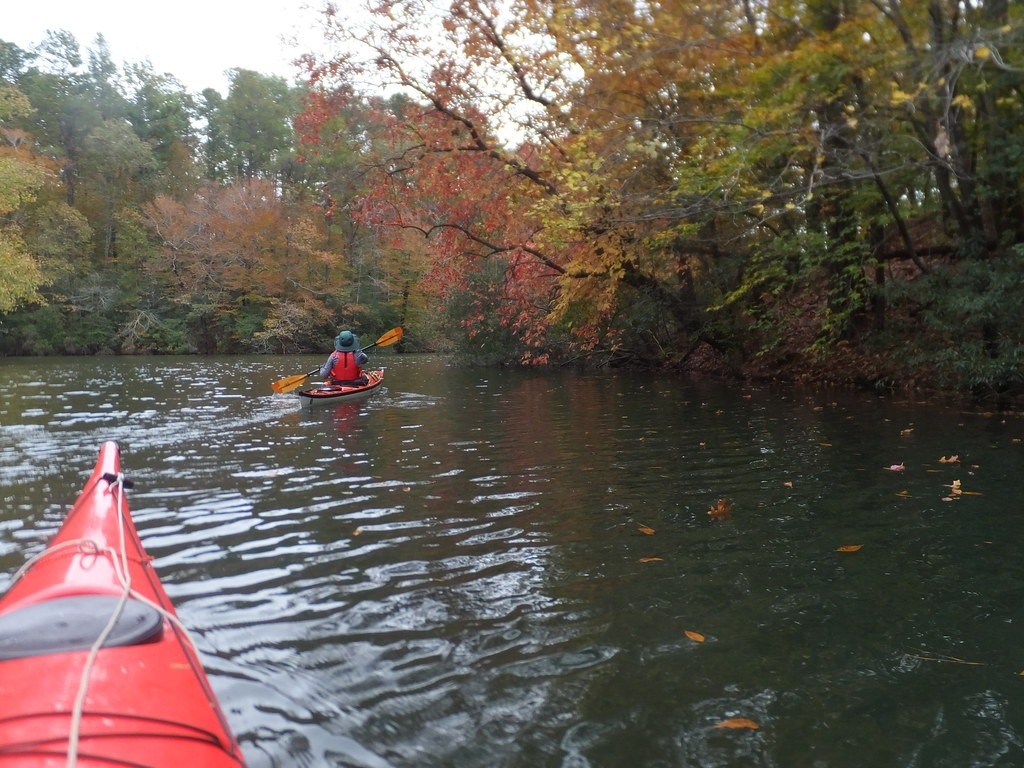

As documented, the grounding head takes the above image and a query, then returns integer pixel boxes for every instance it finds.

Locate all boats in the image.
[299,366,386,407]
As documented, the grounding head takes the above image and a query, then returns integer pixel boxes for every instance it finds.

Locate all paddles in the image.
[271,326,403,393]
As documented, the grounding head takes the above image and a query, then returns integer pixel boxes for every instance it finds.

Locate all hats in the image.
[333,329,361,352]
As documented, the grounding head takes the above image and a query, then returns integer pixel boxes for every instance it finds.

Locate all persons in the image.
[319,330,368,386]
